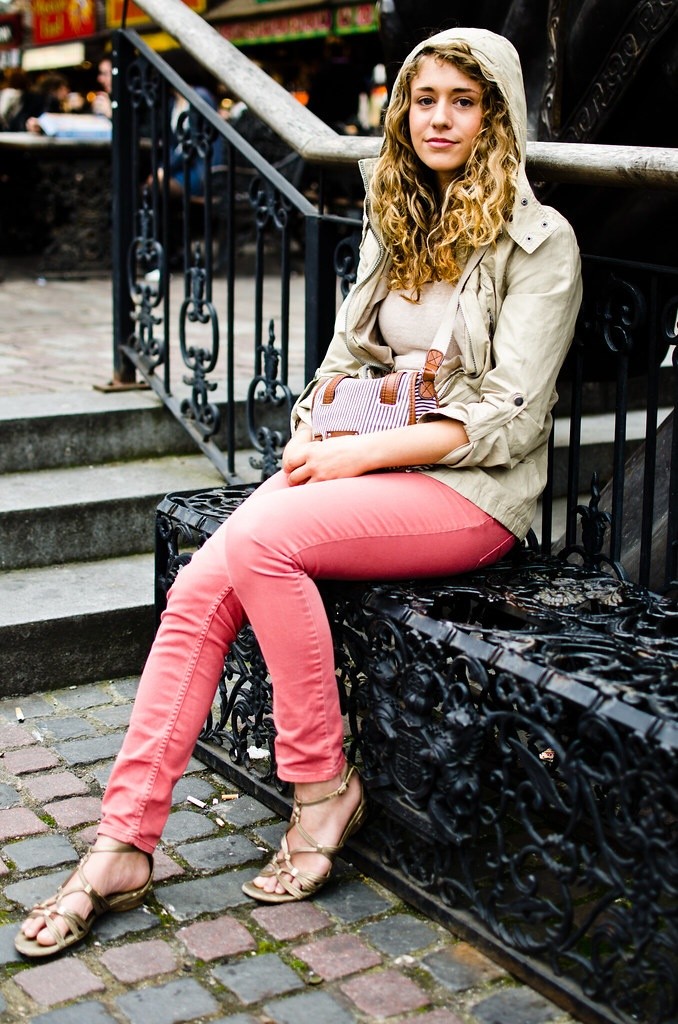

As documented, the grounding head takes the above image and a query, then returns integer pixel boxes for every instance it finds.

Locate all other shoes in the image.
[143,269,160,283]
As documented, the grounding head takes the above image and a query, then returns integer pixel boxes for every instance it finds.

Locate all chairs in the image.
[186,151,307,279]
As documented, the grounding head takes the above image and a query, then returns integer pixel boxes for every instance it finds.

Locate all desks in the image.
[0,132,170,283]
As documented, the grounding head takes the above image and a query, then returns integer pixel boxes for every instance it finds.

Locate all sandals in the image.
[241,767,365,903]
[13,843,155,956]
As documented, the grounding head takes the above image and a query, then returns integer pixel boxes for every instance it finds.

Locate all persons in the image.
[0,54,300,274]
[13,29,583,955]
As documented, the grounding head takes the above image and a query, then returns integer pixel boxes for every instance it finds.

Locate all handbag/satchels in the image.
[309,368,441,473]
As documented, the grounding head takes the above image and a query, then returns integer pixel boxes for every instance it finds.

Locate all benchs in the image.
[155,488,678,1023]
